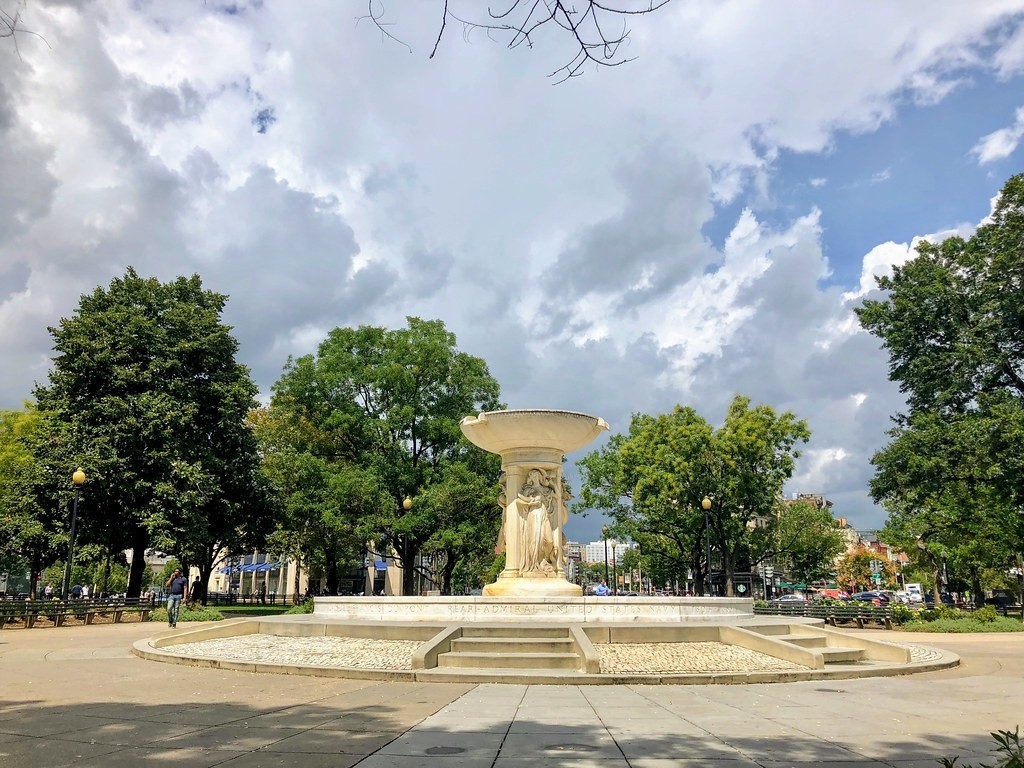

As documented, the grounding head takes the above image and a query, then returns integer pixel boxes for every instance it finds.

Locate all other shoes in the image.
[172,624,176,629]
[168,622,172,627]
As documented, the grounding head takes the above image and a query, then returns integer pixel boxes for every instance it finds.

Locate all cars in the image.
[895,584,924,603]
[773,595,804,603]
[842,589,904,605]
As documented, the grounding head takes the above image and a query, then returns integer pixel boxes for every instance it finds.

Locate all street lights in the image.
[702,496,713,595]
[602,524,609,585]
[61,466,85,605]
[610,541,617,596]
[402,495,413,595]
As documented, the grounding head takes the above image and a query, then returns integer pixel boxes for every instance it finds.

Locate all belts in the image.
[173,593,178,595]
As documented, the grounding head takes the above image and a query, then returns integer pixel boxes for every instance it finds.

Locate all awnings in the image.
[215,562,280,574]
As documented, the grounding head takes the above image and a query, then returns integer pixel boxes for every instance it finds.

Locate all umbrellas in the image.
[777,581,812,590]
[820,583,837,589]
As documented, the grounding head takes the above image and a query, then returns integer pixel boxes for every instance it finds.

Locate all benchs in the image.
[751,606,893,630]
[0,598,151,629]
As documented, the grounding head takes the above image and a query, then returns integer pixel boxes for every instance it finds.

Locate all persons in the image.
[952,590,959,604]
[321,589,328,596]
[44,582,53,595]
[142,587,156,604]
[189,575,200,601]
[123,591,126,602]
[260,581,266,605]
[166,567,187,628]
[965,588,970,603]
[71,582,83,599]
[254,588,258,595]
[596,579,611,596]
[517,468,554,573]
[114,591,119,597]
[83,584,89,599]
